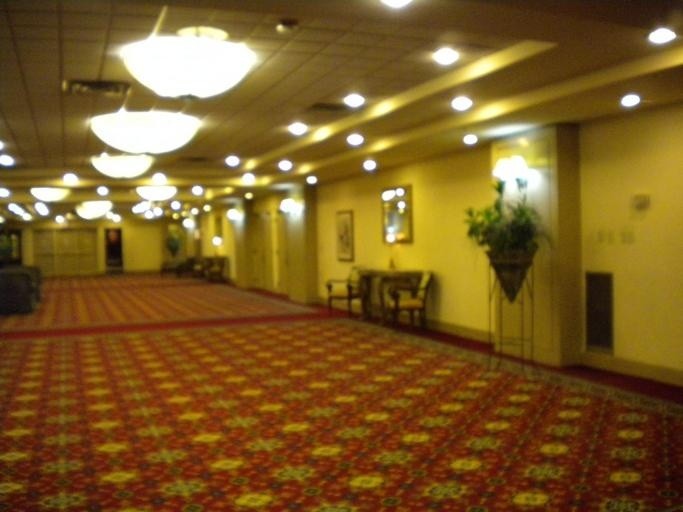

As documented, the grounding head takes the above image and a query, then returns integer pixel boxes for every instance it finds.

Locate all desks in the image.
[357,268,423,322]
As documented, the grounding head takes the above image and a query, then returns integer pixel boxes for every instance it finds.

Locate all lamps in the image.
[114,30,260,102]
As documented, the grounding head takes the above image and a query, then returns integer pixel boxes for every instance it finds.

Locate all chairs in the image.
[324,262,368,319]
[383,266,432,330]
[175,253,231,285]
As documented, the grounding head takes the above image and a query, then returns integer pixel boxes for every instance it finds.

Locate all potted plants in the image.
[460,175,559,306]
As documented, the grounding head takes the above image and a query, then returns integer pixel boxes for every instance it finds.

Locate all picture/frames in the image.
[334,207,355,264]
[379,180,414,247]
